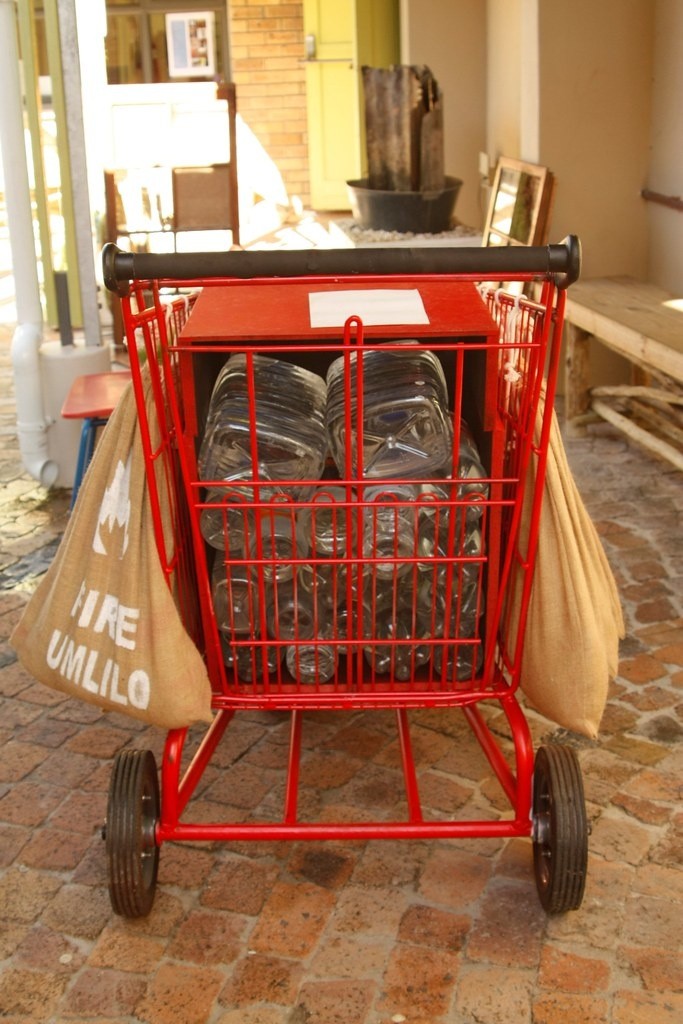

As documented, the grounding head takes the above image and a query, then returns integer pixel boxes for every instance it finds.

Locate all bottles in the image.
[198,338,487,687]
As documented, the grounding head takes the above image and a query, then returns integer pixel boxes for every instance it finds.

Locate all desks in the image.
[535,274,683,472]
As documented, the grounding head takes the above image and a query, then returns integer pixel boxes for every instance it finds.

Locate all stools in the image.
[59,373,132,508]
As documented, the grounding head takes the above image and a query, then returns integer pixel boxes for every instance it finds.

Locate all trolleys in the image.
[101,235,595,913]
